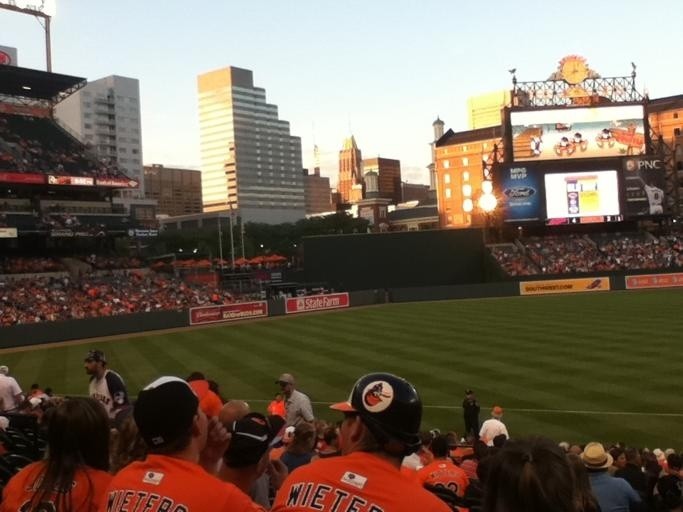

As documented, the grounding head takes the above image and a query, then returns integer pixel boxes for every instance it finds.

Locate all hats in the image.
[223,412,271,468]
[282,425,298,445]
[327,372,423,438]
[493,434,506,447]
[466,388,474,395]
[273,372,298,389]
[84,348,109,365]
[491,405,506,419]
[653,446,676,462]
[579,441,615,470]
[132,375,201,448]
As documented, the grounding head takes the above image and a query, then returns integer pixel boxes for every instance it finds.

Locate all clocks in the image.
[561,59,589,85]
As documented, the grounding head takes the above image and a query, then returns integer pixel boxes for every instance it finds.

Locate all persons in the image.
[0,112,119,180]
[639,176,665,214]
